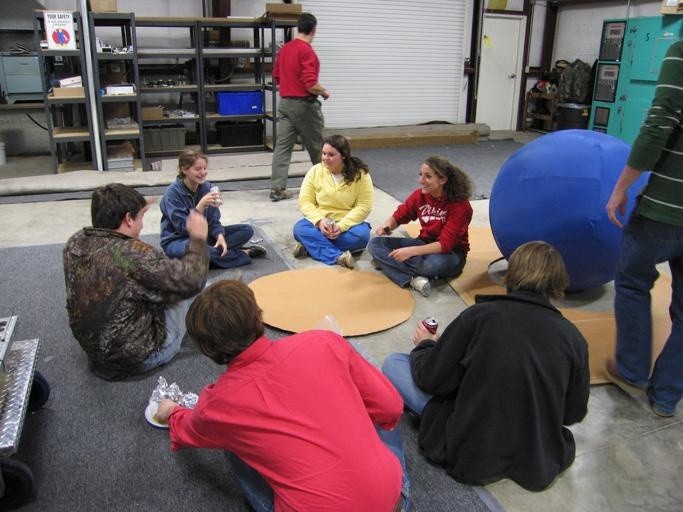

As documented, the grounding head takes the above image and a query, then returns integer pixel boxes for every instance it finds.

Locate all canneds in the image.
[210,186,223,207]
[326,219,334,238]
[422,317,438,334]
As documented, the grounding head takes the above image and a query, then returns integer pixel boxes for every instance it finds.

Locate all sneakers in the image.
[337,249,355,268]
[293,243,308,257]
[269,189,292,200]
[410,276,432,297]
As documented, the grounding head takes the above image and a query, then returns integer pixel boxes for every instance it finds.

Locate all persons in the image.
[381,241,589,492]
[369,157,474,296]
[155,281,412,512]
[63,184,210,383]
[606,41,683,416]
[160,149,266,269]
[269,14,331,202]
[292,135,374,269]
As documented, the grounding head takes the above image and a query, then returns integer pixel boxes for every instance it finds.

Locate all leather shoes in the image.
[646,380,675,418]
[603,355,646,400]
[238,246,268,259]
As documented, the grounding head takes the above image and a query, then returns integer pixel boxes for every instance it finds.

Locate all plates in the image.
[145,400,171,429]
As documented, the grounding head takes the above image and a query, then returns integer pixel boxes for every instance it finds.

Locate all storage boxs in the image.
[87,0,117,12]
[52,87,86,98]
[215,91,265,115]
[136,126,187,152]
[658,0,683,15]
[106,84,134,95]
[214,121,265,148]
[265,2,302,21]
[141,104,165,120]
[98,61,128,84]
[56,74,83,88]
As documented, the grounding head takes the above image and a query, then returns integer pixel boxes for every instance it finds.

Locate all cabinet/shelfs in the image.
[127,21,201,159]
[201,25,269,152]
[31,8,98,172]
[260,19,303,151]
[522,90,558,134]
[0,0,44,105]
[87,10,144,172]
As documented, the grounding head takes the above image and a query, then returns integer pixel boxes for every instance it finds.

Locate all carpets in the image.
[0,219,501,511]
